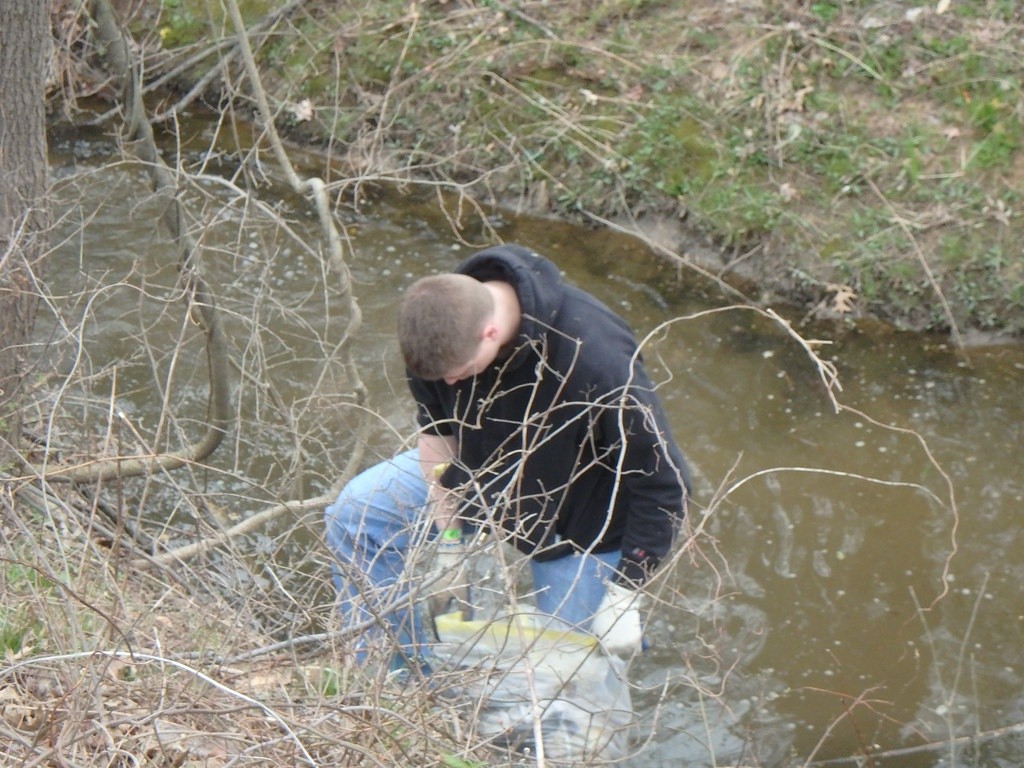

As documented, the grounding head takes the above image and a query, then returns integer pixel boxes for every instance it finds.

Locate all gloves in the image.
[425,542,473,643]
[590,579,645,660]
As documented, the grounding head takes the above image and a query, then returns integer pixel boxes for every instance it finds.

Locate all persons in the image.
[324,244,692,694]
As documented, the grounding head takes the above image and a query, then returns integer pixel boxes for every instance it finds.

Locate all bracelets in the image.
[440,529,462,540]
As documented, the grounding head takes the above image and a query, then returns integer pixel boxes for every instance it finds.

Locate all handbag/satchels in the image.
[430,608,634,765]
